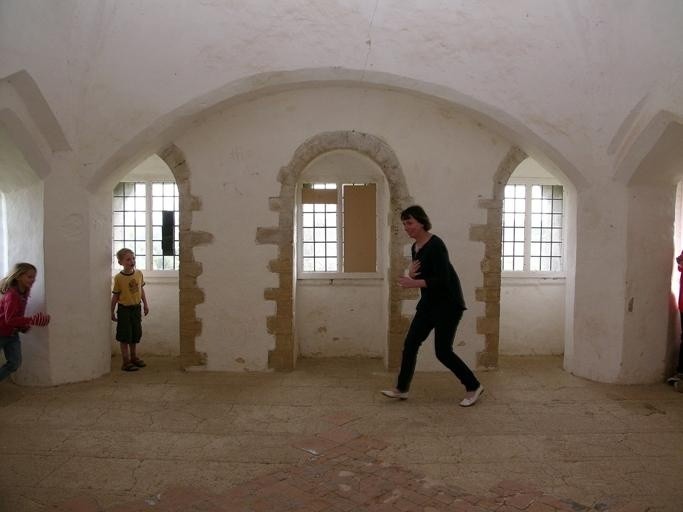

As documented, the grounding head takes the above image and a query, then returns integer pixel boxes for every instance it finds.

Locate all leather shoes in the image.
[381,389,409,399]
[460,384,484,406]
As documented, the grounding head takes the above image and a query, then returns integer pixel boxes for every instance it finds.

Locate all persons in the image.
[664,249,683,393]
[108,247,148,370]
[378,205,484,407]
[0,263,50,379]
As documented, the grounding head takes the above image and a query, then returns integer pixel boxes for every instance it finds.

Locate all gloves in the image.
[31,311,51,327]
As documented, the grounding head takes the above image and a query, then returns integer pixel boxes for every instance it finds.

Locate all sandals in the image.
[122,357,146,371]
